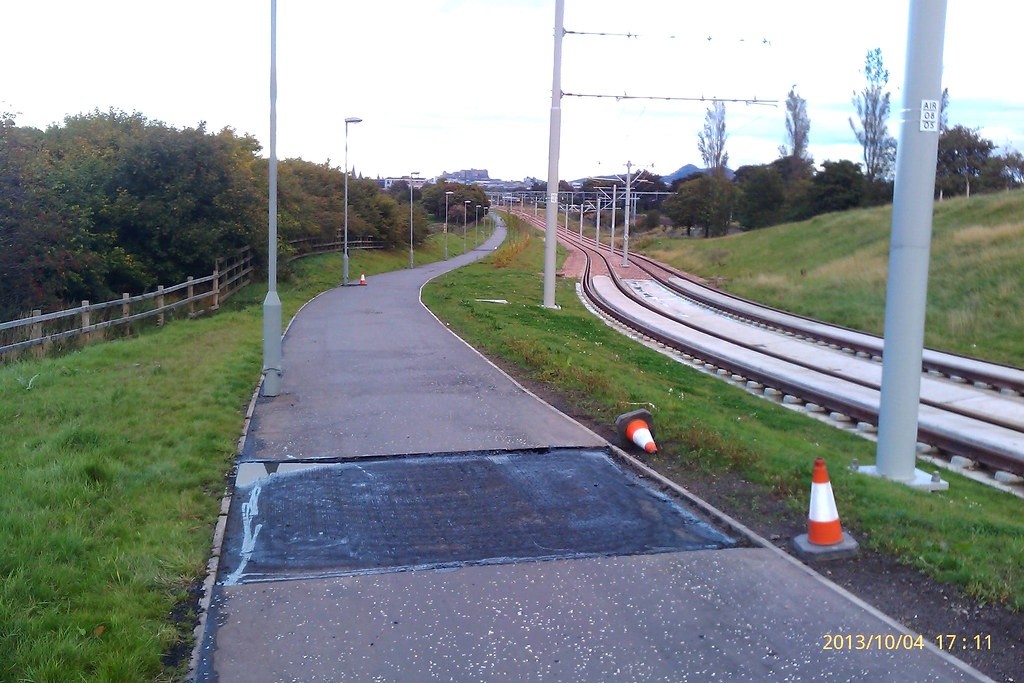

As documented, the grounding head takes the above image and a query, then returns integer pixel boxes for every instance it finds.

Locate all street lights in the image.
[340,117,363,282]
[484,207,488,227]
[463,201,471,254]
[410,172,419,268]
[445,192,454,261]
[475,205,481,246]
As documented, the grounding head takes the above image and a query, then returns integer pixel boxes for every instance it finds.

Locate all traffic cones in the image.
[358,273,367,286]
[617,409,658,454]
[794,457,859,561]
